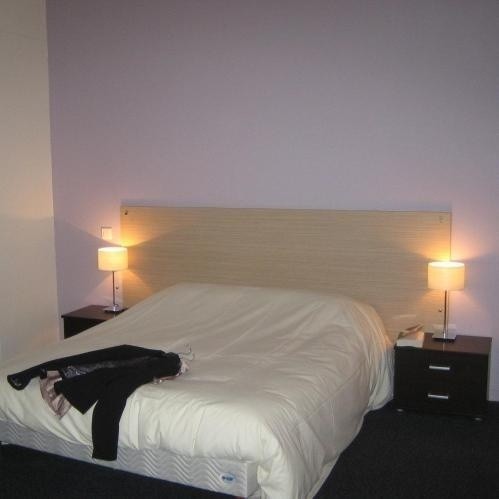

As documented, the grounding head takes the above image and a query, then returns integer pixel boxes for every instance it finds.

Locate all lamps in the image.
[427,261,465,340]
[97,247,128,312]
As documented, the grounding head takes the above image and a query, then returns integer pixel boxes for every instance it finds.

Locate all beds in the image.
[0,205,452,499]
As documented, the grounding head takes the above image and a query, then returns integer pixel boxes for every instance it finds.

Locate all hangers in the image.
[176,344,195,362]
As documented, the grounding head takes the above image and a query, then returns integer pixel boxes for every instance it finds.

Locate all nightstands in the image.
[60,305,129,339]
[393,332,492,422]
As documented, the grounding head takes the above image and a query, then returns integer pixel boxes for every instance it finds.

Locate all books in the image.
[395,323,425,349]
